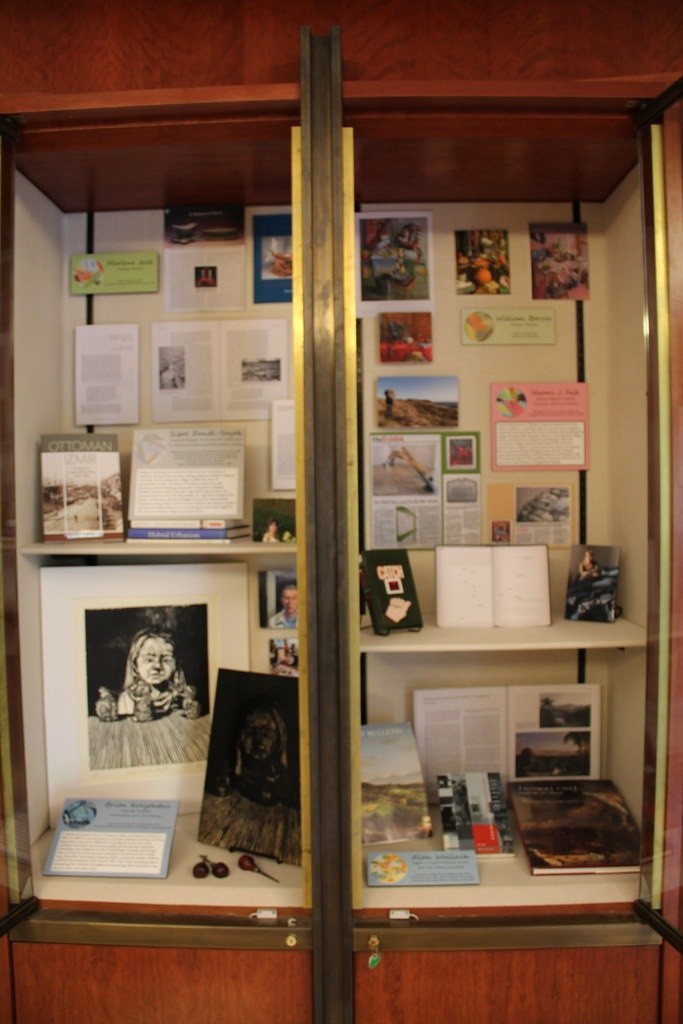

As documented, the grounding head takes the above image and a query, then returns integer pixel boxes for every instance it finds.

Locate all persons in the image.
[260,519,280,543]
[268,582,302,628]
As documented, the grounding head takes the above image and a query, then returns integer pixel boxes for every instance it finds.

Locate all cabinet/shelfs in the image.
[0,0,683,1024]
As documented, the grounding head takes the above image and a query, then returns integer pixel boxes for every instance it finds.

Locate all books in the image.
[432,540,555,631]
[39,431,130,548]
[503,777,644,878]
[362,548,426,638]
[124,423,246,524]
[435,769,515,861]
[411,681,604,798]
[196,665,304,875]
[360,718,427,853]
[124,518,252,545]
[562,540,621,623]
